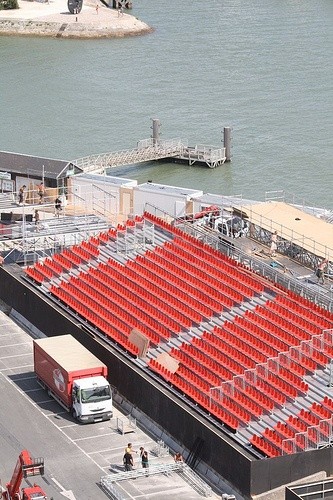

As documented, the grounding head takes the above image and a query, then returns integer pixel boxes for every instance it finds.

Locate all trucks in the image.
[33,333,114,424]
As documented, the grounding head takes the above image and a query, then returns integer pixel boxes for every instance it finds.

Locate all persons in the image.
[174,451,183,462]
[54,196,62,217]
[269,229,279,256]
[18,184,26,203]
[317,257,329,284]
[139,447,149,469]
[123,448,134,471]
[210,212,216,228]
[34,210,39,225]
[125,443,133,454]
[39,182,44,201]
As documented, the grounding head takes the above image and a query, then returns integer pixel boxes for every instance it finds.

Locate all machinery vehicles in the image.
[0,449,54,500]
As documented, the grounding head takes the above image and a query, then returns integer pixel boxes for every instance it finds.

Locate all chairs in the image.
[24,211,333,457]
